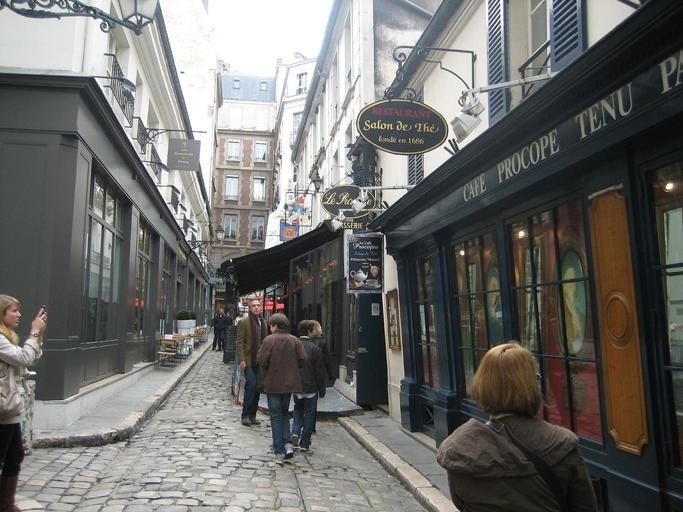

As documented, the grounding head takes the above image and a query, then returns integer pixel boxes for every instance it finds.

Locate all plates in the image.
[363,282,382,289]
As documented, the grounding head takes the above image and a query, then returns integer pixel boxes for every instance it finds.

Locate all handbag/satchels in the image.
[1,375,22,415]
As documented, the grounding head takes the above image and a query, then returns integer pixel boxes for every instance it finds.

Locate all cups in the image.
[366,279,381,287]
[370,268,380,278]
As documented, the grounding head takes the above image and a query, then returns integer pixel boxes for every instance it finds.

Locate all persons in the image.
[390,307,396,346]
[256,313,307,465]
[486,278,503,328]
[435,340,602,510]
[235,300,270,427]
[0,295,47,512]
[310,320,336,435]
[563,267,587,354]
[210,306,246,362]
[290,320,324,451]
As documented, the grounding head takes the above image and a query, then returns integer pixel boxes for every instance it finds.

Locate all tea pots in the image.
[350,268,368,284]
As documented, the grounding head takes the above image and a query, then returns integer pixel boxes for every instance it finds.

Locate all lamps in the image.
[331,185,414,233]
[187,224,224,249]
[0,0,158,35]
[449,71,559,144]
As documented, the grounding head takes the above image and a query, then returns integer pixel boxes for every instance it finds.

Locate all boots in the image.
[1,475,22,512]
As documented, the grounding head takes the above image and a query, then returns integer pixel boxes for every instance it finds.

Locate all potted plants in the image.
[176,309,196,349]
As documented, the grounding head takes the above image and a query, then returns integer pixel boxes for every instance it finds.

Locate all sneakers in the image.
[272,434,307,464]
[242,417,261,425]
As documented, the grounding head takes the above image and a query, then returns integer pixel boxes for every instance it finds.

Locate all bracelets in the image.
[30,328,39,337]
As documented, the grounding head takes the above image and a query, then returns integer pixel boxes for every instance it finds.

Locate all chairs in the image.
[156,328,203,369]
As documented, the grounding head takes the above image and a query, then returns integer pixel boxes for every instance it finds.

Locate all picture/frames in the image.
[385,288,401,351]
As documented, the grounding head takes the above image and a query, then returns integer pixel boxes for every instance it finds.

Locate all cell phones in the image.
[42,305,46,313]
